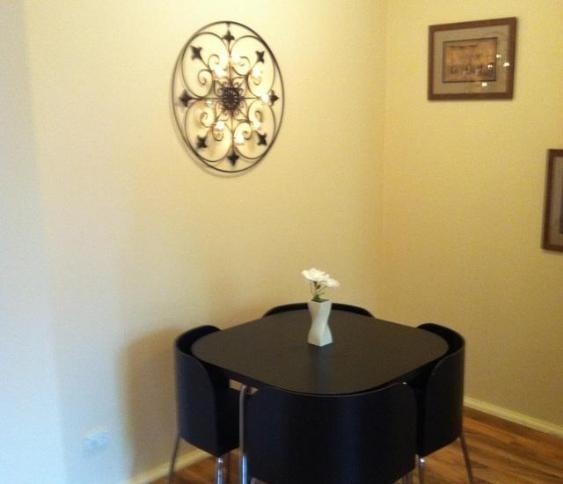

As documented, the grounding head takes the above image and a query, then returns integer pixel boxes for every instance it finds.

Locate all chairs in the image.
[408,322,472,484]
[168,323,241,484]
[244,380,414,484]
[263,302,376,317]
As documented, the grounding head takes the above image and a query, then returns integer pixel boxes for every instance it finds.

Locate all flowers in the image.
[302,266,336,300]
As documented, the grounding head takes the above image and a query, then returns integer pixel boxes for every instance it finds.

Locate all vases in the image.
[306,301,333,348]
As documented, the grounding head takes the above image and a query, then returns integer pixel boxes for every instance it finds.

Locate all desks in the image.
[188,307,450,397]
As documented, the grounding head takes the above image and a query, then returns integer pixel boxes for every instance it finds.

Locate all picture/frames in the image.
[428,17,516,100]
[541,149,563,252]
